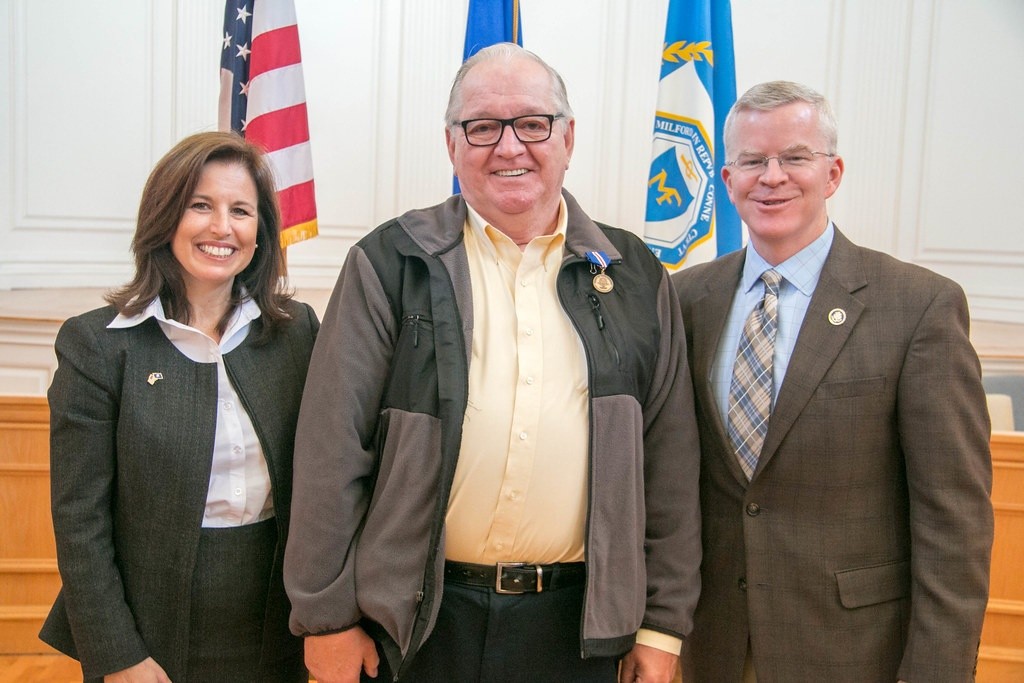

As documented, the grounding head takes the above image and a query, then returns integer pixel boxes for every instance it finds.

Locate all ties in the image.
[726,268,783,485]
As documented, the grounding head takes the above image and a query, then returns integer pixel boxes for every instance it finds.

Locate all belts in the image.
[442,558,589,600]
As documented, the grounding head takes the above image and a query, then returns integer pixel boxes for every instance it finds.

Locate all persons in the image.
[38,131,320,683]
[671,82,994,683]
[282,42,701,683]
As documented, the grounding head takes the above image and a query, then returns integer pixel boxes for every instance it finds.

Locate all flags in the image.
[453,0,523,192]
[218,0,318,249]
[645,0,743,274]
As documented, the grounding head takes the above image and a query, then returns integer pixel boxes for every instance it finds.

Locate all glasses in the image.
[452,113,566,148]
[724,146,837,175]
